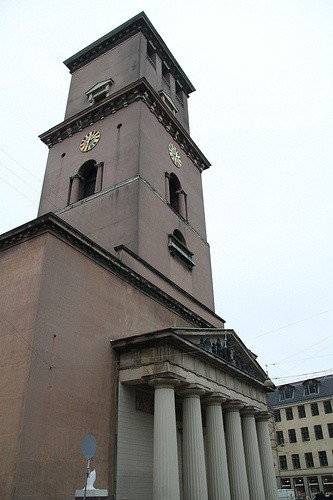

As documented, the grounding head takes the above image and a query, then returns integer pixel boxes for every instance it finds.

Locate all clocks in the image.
[167,143,181,168]
[79,130,99,153]
